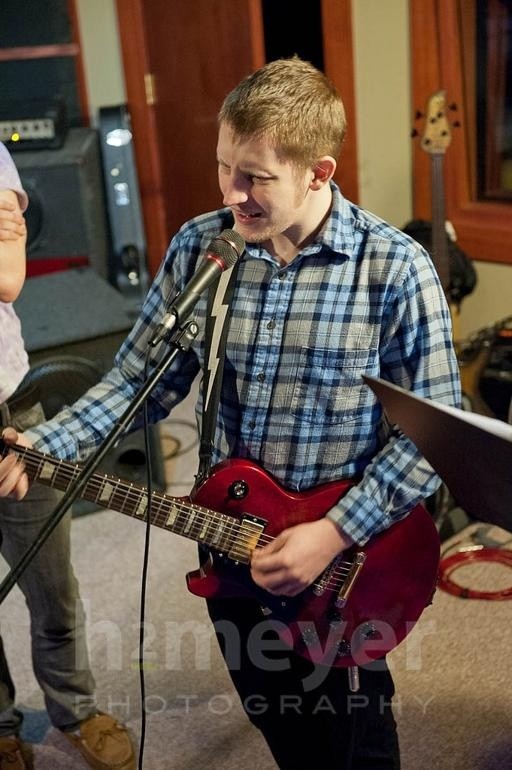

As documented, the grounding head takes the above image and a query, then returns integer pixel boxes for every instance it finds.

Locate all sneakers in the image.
[0,731,35,770]
[62,708,137,770]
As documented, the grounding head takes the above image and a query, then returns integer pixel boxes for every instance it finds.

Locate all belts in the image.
[0,383,40,427]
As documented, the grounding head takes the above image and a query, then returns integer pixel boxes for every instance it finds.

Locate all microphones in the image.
[149,228,247,347]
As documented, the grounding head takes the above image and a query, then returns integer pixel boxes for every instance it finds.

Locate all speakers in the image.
[9,264,166,518]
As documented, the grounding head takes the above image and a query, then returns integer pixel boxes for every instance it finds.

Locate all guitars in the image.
[400,89,476,316]
[0,440,440,668]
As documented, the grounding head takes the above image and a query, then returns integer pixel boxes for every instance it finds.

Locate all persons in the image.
[1,53,468,770]
[1,135,140,768]
